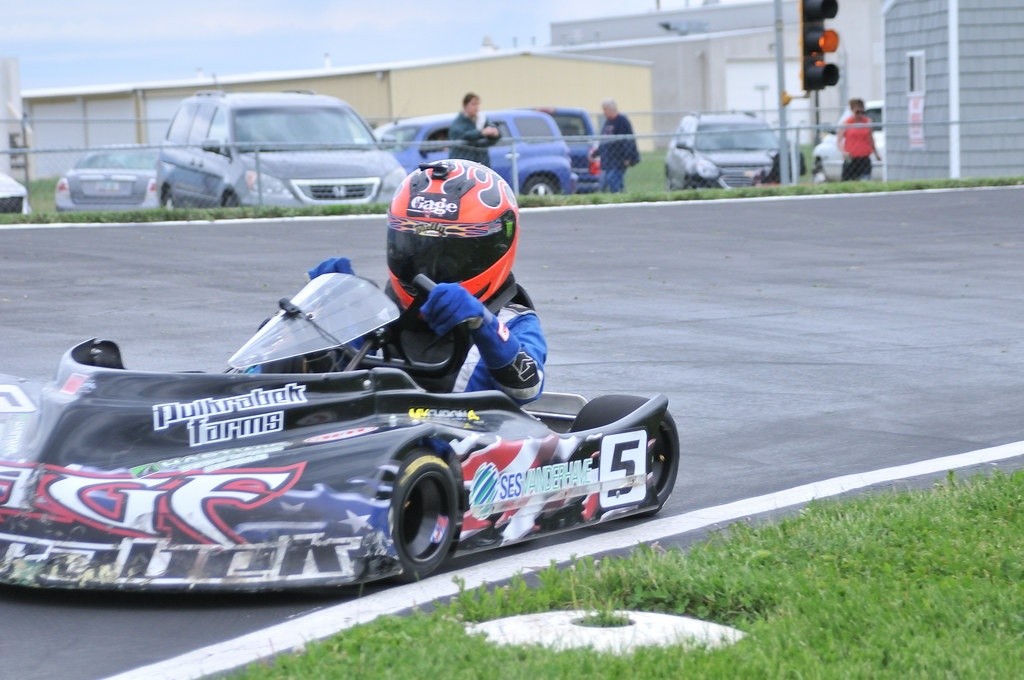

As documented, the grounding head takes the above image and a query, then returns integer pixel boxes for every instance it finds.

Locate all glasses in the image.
[856,110,866,115]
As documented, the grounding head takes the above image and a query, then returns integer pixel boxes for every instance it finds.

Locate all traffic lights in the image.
[799,0,839,90]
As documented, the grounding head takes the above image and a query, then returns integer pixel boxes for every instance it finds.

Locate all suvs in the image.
[156,89,407,209]
[665,110,782,191]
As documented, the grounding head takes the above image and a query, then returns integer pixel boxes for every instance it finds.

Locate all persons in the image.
[239,159,548,404]
[588,99,641,193]
[837,99,881,180]
[449,93,502,168]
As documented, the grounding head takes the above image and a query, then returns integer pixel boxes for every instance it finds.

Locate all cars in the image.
[372,109,578,196]
[54,151,156,214]
[812,100,884,182]
[538,108,603,195]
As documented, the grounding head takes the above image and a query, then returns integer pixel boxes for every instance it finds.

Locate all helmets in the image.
[386,157,520,323]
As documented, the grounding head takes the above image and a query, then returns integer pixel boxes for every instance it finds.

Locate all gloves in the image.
[419,282,521,370]
[308,257,376,373]
